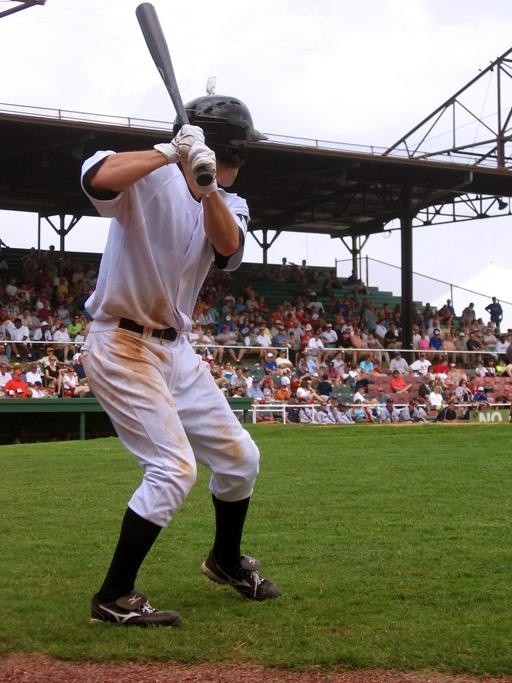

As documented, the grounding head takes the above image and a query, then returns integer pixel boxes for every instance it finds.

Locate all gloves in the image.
[186,141,219,196]
[154,124,207,162]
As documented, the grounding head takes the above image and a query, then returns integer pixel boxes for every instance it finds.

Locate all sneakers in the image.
[90,591,183,628]
[200,548,282,601]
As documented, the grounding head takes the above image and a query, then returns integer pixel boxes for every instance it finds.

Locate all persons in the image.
[2,245,512,425]
[81,94,282,628]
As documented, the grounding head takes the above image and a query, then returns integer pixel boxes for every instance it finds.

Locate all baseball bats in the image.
[134,3,213,186]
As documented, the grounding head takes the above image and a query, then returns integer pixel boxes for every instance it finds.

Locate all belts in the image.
[118,315,177,343]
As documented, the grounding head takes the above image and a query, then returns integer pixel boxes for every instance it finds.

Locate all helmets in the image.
[174,94,268,168]
